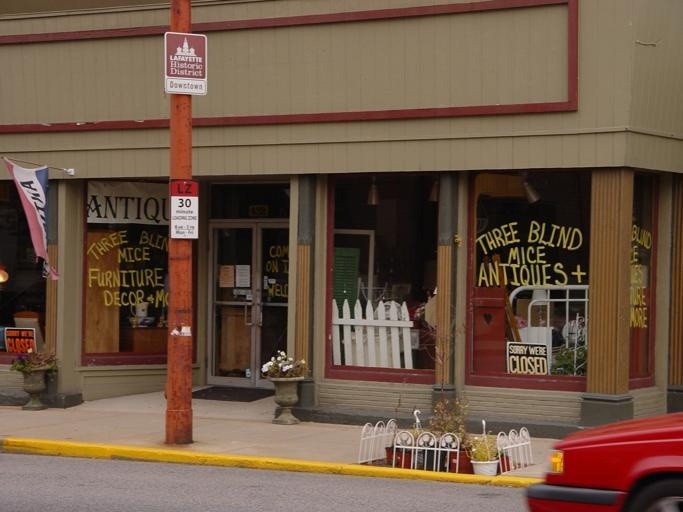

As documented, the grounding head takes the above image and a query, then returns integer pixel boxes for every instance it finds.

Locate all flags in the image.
[3,157,60,281]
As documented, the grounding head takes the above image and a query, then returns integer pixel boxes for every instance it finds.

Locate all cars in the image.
[525,411,683,512]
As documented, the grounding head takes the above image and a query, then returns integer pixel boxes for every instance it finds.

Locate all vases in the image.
[384,445,510,476]
[19,368,49,412]
[268,377,303,427]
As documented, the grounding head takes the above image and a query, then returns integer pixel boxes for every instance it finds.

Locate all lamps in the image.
[366,176,382,207]
[522,173,542,208]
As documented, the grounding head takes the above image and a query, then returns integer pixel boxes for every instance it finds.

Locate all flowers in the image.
[397,394,501,460]
[258,348,307,377]
[6,343,59,374]
